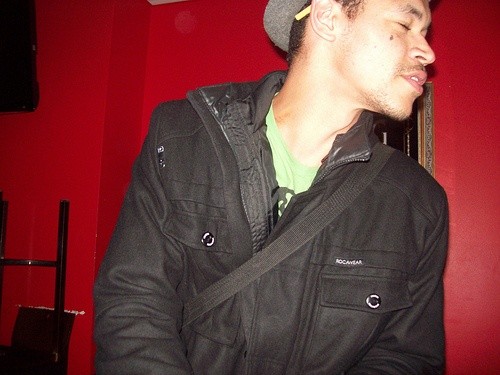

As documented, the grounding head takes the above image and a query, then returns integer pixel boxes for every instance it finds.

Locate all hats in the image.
[264,0,308,51]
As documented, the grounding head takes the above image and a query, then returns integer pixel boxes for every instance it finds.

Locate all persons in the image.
[94,0,449,375]
